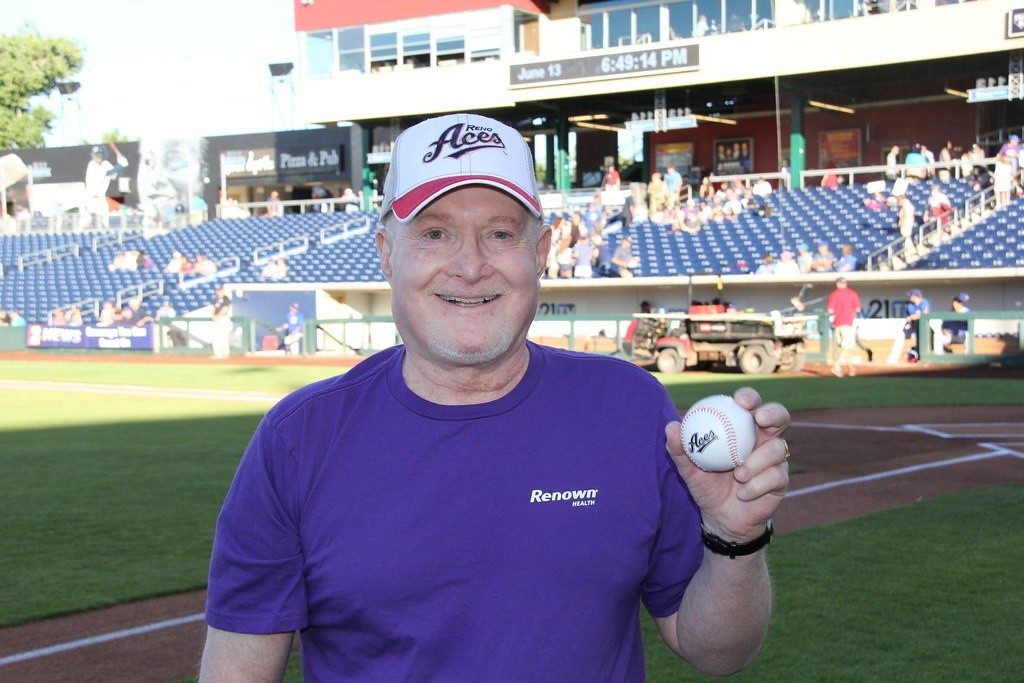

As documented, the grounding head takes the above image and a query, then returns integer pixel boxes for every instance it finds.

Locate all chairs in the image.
[0,172,1024,351]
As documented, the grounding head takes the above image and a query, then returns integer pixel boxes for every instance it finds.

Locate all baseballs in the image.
[681,393,757,472]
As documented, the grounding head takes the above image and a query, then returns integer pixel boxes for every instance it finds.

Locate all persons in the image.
[1,299,176,327]
[754,242,856,273]
[86,143,128,230]
[886,134,1024,238]
[137,137,209,220]
[782,159,789,190]
[717,142,747,163]
[210,285,231,356]
[600,166,620,186]
[612,236,639,278]
[827,277,862,378]
[628,166,773,235]
[544,195,607,280]
[821,164,845,187]
[942,293,970,351]
[261,258,287,278]
[278,302,305,354]
[265,190,283,215]
[108,250,216,276]
[698,15,708,36]
[194,113,790,683]
[622,190,638,227]
[902,289,929,339]
[342,188,359,212]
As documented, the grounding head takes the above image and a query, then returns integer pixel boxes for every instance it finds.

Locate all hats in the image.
[796,243,809,252]
[1010,135,1019,143]
[818,241,830,245]
[906,289,922,298]
[956,293,969,304]
[379,113,543,228]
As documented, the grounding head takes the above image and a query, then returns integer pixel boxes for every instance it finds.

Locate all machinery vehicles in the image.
[605,302,825,377]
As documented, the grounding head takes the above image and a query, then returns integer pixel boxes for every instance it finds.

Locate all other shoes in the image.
[849,370,856,377]
[830,368,842,378]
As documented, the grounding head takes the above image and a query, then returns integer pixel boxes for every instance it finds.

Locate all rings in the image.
[782,439,790,462]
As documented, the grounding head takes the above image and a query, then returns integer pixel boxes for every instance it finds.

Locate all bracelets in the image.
[702,518,774,559]
